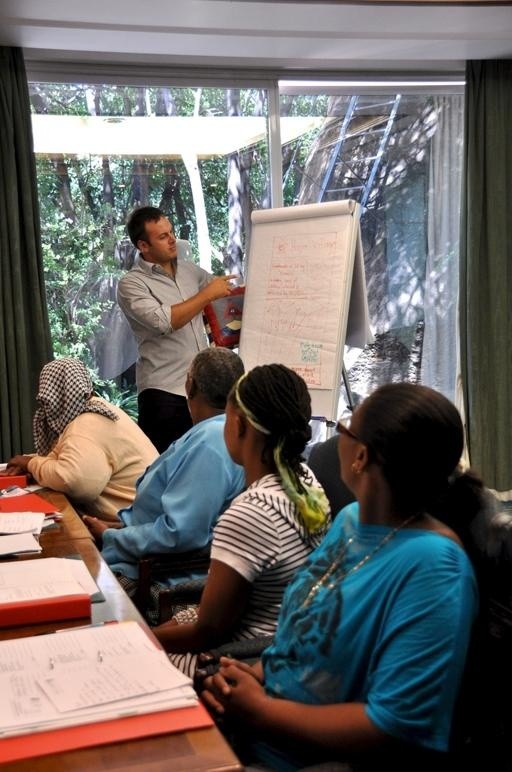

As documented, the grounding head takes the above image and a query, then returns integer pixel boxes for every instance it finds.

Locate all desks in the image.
[1,540,245,771]
[1,479,98,545]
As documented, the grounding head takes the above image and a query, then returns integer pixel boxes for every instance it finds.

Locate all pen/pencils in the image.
[30,619,119,638]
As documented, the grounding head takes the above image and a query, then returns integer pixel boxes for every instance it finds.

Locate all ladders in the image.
[316,93,402,211]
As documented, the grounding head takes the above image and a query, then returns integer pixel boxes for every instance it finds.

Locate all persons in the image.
[200,381,495,770]
[7,356,160,534]
[151,364,332,686]
[114,205,243,456]
[79,344,243,626]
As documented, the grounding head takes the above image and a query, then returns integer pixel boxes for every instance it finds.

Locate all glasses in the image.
[335,399,366,445]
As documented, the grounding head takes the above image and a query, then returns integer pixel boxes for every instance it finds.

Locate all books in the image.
[0,555,104,609]
[0,621,214,762]
[0,462,65,534]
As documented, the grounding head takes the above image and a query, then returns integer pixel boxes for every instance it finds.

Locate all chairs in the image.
[223,482,511,772]
[134,555,212,626]
[136,389,195,456]
[157,437,359,689]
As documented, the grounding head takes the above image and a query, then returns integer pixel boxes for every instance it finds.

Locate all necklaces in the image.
[295,510,420,611]
